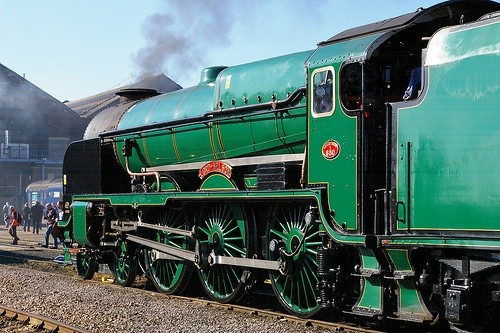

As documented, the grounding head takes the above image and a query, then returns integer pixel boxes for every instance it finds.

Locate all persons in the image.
[2,202,11,228]
[22,203,31,232]
[30,201,45,234]
[42,201,64,249]
[5,206,22,245]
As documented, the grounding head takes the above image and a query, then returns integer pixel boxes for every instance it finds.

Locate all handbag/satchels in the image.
[12,220,18,226]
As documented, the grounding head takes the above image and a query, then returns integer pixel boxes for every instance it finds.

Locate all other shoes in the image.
[50,246,57,249]
[11,242,17,244]
[41,245,48,248]
[16,237,19,240]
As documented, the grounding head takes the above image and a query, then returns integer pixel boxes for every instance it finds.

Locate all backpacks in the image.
[18,213,22,223]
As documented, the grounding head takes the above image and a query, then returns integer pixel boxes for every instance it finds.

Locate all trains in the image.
[24,178,63,221]
[52,0,500,333]
